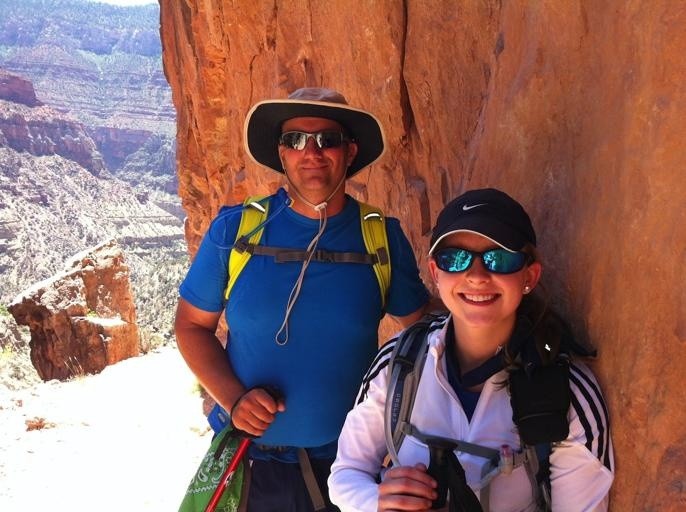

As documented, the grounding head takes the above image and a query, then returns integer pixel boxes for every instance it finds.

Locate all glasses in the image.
[277,129,354,151]
[432,246,528,274]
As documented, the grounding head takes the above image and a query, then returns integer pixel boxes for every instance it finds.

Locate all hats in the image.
[429,188,538,256]
[242,87,387,179]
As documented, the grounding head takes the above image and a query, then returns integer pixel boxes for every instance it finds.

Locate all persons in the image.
[174,87,434,511]
[327,188,615,512]
